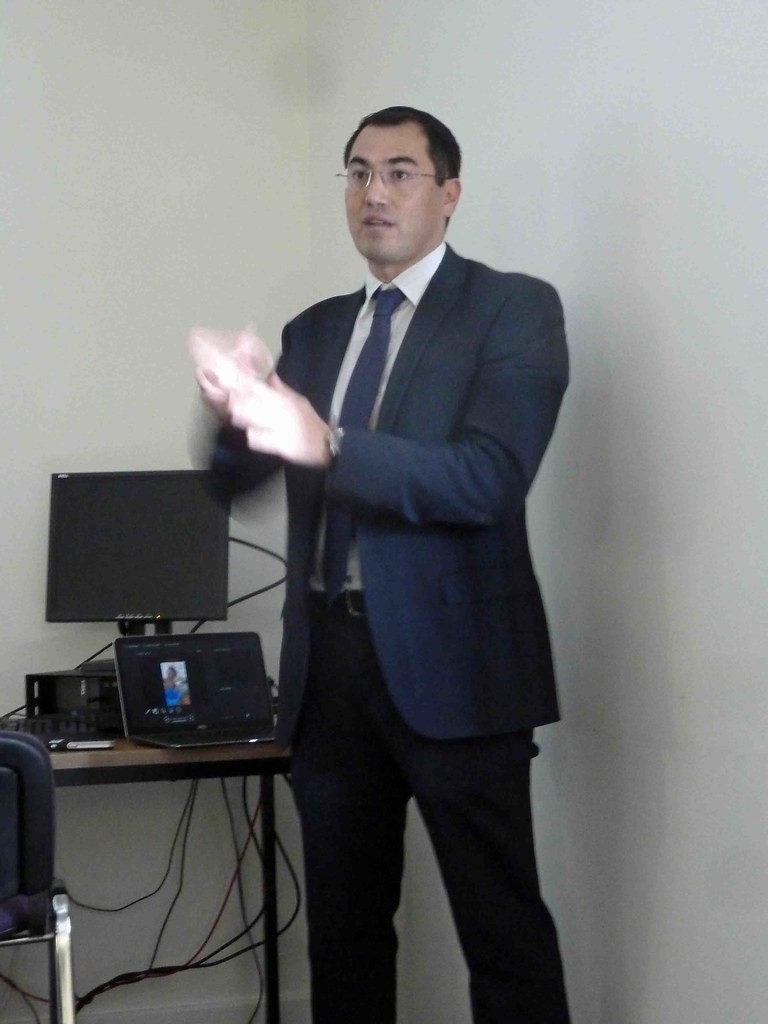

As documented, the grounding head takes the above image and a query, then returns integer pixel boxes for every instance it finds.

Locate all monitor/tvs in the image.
[41,469,231,672]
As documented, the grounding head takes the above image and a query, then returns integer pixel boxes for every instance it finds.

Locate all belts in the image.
[310,591,368,621]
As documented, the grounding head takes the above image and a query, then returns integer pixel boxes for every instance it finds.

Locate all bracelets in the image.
[325,428,345,457]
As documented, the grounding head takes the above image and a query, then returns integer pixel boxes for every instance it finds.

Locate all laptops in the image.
[111,630,276,748]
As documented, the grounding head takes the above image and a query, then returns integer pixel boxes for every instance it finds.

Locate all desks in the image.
[50,740,294,1024]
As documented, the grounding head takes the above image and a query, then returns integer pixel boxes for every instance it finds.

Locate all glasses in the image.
[337,168,448,189]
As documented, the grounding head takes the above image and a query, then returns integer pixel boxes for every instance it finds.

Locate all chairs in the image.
[0,730,75,1024]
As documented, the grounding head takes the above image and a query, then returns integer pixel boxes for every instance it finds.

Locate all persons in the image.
[192,106,575,1024]
[163,666,181,704]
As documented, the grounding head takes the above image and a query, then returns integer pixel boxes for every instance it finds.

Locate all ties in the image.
[321,288,406,606]
